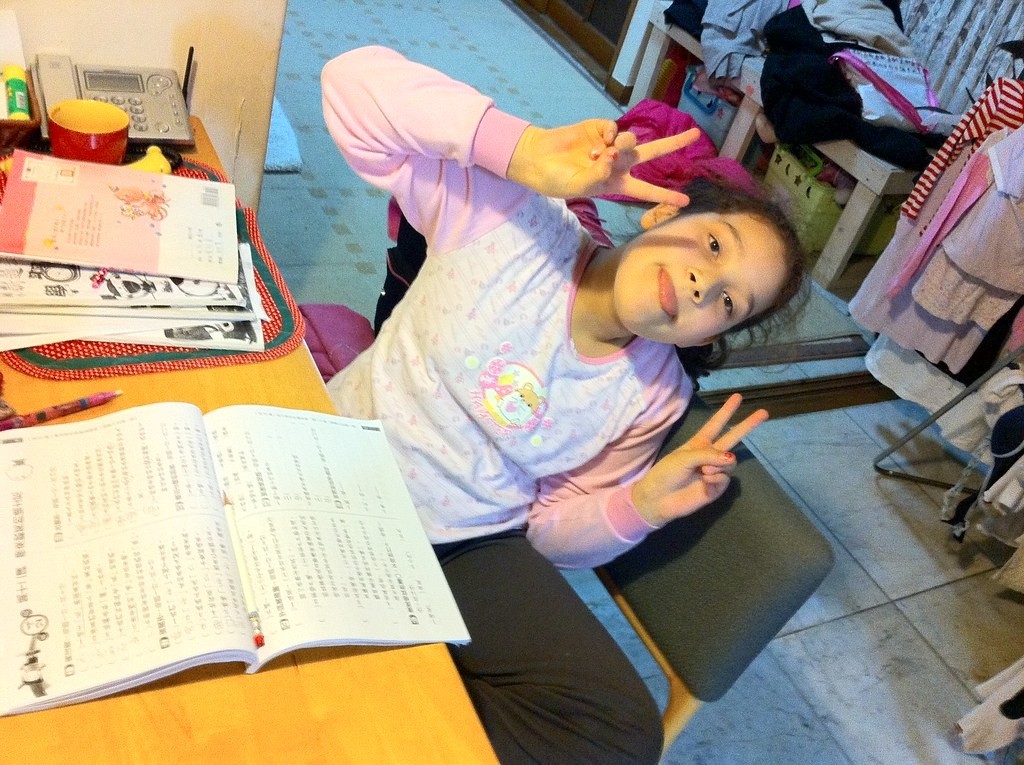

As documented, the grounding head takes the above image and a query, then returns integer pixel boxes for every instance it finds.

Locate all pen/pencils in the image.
[0,389,123,432]
[222,491,265,647]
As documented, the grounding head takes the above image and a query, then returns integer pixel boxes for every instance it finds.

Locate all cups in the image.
[47,98,130,167]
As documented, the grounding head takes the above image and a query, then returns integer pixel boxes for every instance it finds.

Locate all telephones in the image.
[30,46,195,151]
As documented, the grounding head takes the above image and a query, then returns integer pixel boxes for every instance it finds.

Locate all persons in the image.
[321,46,806,765]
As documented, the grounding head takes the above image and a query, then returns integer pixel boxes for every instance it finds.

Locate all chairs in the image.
[595,393,835,755]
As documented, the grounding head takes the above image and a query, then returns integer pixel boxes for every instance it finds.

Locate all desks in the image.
[0,115,500,765]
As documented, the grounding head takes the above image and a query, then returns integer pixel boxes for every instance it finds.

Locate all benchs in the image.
[628,0,935,293]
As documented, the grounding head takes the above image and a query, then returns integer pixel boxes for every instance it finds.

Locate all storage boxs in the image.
[764,143,900,256]
[678,64,738,150]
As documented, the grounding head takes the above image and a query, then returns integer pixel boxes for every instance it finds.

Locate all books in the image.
[0,149,269,353]
[2,401,470,716]
